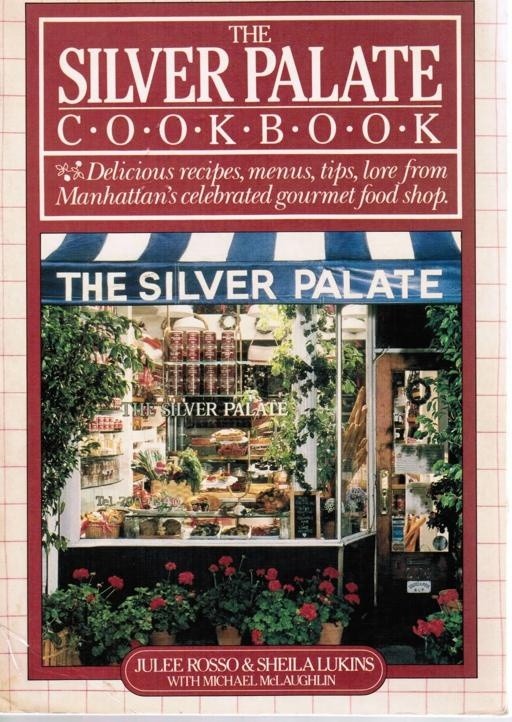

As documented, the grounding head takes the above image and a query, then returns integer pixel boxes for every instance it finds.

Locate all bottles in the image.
[80,458,121,487]
[112,434,117,455]
[135,497,141,508]
[115,418,123,430]
[278,511,291,540]
[134,413,142,430]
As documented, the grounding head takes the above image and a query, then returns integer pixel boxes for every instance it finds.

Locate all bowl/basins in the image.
[216,432,246,441]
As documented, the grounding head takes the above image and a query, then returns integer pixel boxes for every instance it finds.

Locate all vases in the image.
[215,624,243,647]
[148,627,176,649]
[316,622,348,648]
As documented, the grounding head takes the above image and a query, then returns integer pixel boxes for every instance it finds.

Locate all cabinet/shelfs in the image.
[80,304,122,510]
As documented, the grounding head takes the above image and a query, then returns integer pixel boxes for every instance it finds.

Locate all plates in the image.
[250,525,279,539]
[219,523,252,540]
[189,522,221,540]
[136,527,185,538]
[129,503,155,513]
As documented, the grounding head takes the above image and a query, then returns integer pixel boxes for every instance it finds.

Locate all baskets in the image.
[85,523,122,538]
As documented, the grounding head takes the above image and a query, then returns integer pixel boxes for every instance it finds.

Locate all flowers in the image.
[55,552,465,658]
